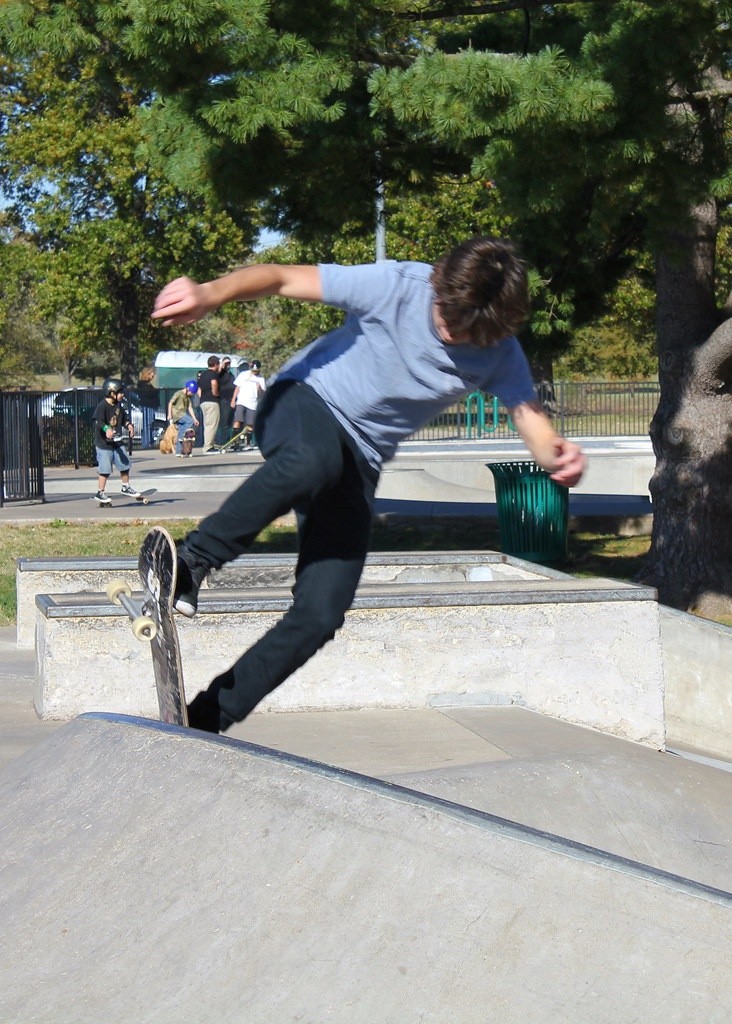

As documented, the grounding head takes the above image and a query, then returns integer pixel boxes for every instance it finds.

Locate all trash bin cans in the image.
[485,460,571,570]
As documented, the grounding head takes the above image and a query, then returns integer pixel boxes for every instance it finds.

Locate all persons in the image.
[92,380,140,504]
[137,356,266,457]
[151,236,590,735]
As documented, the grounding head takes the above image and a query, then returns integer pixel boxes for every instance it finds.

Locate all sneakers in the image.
[94,490,112,503]
[120,483,142,497]
[173,537,218,618]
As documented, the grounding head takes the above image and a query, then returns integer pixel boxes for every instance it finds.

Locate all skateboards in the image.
[107,525,189,727]
[89,488,158,508]
[180,429,196,458]
[218,426,248,455]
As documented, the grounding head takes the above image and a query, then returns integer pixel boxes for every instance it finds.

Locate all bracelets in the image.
[102,425,109,432]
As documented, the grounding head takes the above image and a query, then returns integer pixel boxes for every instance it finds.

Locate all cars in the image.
[27,387,171,445]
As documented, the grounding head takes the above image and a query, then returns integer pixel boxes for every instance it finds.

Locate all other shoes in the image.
[176,453,182,457]
[204,448,221,454]
[242,445,253,451]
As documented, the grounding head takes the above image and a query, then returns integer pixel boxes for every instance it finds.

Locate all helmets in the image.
[103,379,124,396]
[250,360,261,370]
[186,380,198,393]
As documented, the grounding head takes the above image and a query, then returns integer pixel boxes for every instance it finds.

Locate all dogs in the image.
[159,422,179,454]
